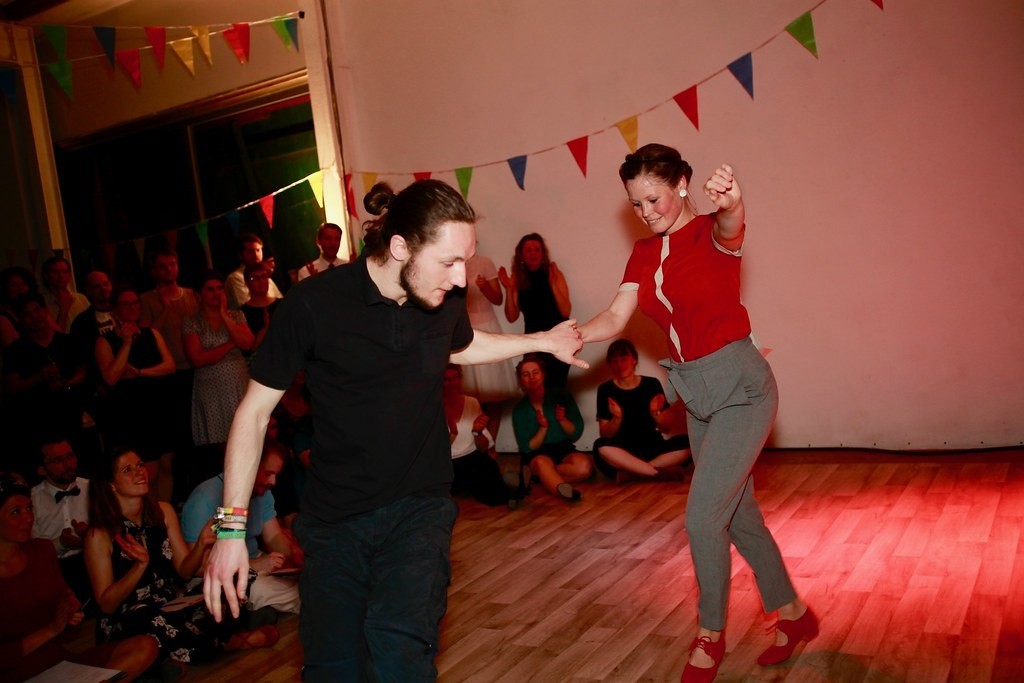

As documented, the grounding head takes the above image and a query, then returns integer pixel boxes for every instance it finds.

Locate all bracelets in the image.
[211,506,249,540]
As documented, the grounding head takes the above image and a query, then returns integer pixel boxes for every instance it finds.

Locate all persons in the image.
[573,142,820,683]
[203,179,590,683]
[0,223,692,683]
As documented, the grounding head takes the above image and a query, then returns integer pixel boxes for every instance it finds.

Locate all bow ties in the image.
[55,486,81,503]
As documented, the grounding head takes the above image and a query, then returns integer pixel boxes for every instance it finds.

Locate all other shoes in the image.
[557,483,581,503]
[615,471,628,485]
[666,466,684,482]
[239,625,280,650]
[160,658,188,680]
[681,626,726,683]
[757,606,819,666]
